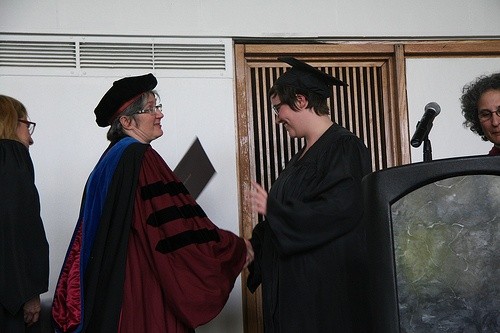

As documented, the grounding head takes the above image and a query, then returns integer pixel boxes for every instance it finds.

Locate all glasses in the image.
[478,107,500,122]
[272,96,297,117]
[126,104,162,116]
[18,119,36,135]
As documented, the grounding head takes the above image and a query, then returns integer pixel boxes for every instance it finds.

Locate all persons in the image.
[459,73,500,156]
[241,56,385,333]
[0,95,49,332]
[51,72,255,333]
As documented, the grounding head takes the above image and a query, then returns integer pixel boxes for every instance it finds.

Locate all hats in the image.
[94,73,157,127]
[273,56,349,97]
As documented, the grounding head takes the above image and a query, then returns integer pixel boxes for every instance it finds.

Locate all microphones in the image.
[411,102,441,147]
